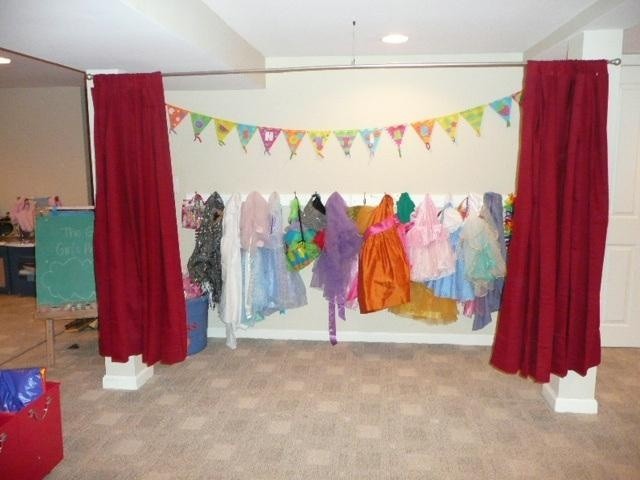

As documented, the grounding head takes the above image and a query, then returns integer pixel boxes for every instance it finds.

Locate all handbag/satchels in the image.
[181,191,209,232]
[285,195,320,272]
[299,192,329,234]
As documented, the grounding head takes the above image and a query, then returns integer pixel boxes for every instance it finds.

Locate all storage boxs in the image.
[0,380,64,480]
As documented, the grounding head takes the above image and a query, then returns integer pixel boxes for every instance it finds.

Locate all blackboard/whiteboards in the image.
[34,205,99,319]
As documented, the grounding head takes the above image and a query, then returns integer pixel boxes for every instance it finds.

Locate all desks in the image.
[0,236,36,296]
